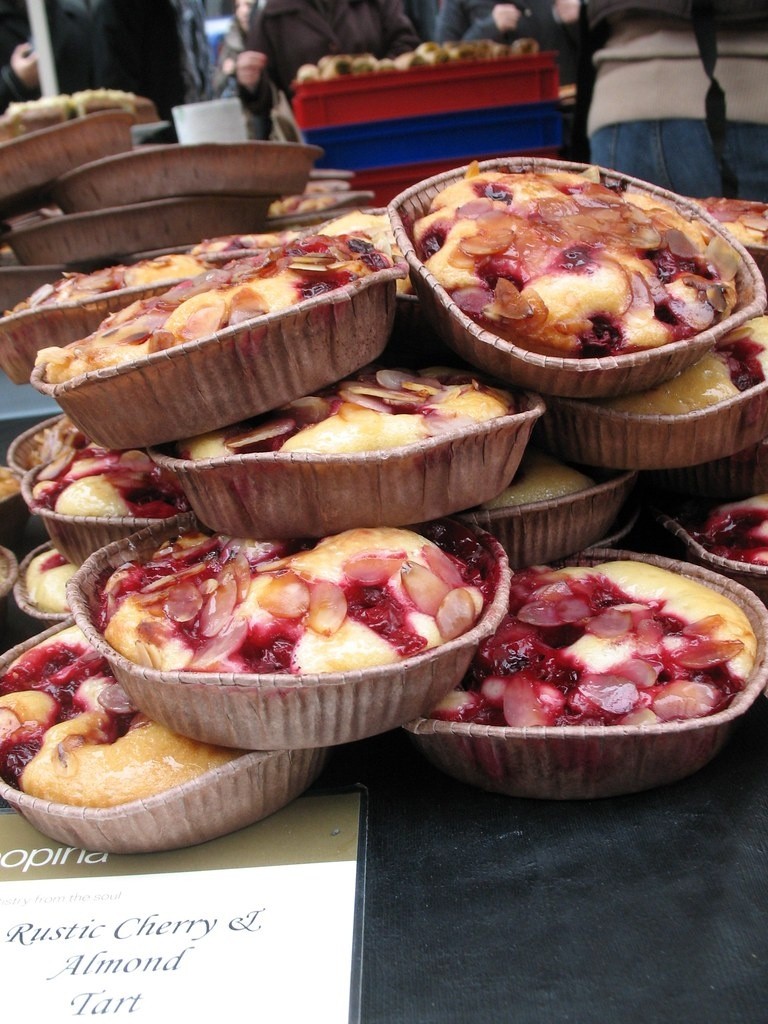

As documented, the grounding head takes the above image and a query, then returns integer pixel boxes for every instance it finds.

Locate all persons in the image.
[0,0,768,206]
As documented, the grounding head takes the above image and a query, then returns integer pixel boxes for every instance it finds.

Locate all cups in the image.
[171,98,249,145]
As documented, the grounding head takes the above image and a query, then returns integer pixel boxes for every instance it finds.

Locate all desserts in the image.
[0,106,768,858]
